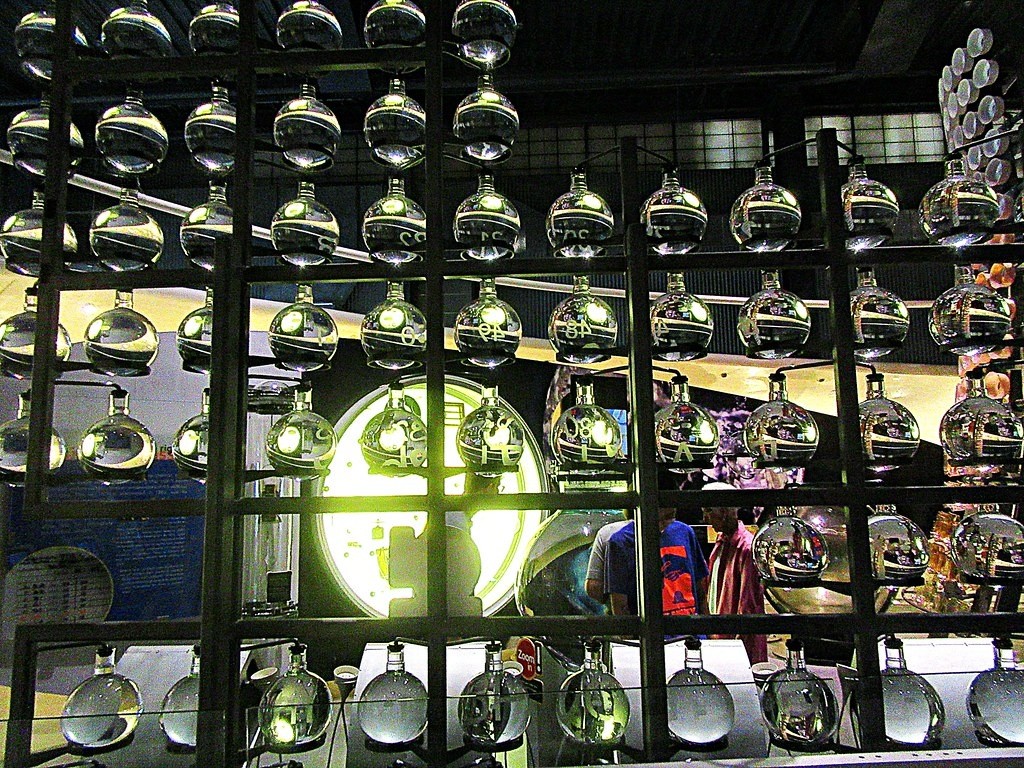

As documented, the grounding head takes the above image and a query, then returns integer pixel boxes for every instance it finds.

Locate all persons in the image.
[587,469,711,643]
[697,482,767,665]
